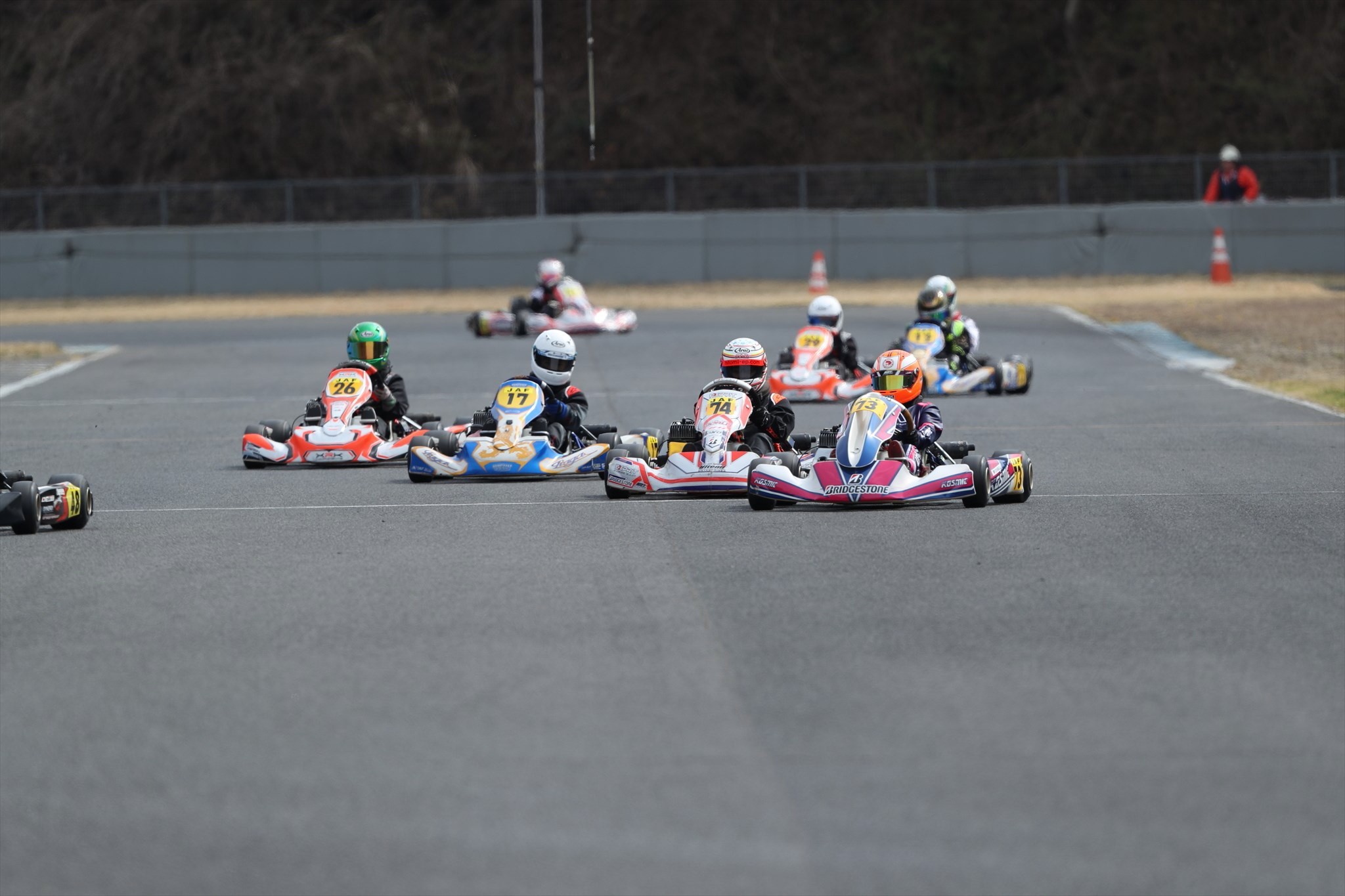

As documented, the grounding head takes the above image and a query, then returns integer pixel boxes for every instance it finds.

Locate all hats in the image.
[1218,144,1240,163]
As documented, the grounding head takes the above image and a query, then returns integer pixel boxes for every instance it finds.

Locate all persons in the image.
[682,336,795,457]
[889,275,979,368]
[778,294,856,379]
[1204,144,1260,202]
[530,257,565,315]
[303,322,410,437]
[481,329,588,450]
[818,350,943,478]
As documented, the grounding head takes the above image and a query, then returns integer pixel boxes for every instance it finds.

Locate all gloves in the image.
[543,397,570,420]
[903,428,925,450]
[749,406,775,428]
[374,383,397,411]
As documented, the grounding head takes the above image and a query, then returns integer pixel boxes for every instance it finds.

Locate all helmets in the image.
[347,321,389,369]
[806,295,844,335]
[925,273,957,310]
[871,349,923,404]
[531,330,578,386]
[719,337,769,393]
[536,258,564,287]
[917,288,949,321]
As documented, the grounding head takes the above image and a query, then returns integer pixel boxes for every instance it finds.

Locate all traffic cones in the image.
[807,250,832,296]
[1210,228,1233,285]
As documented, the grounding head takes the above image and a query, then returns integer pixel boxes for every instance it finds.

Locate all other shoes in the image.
[829,449,836,458]
[532,417,548,436]
[889,440,910,469]
[737,443,752,451]
[682,442,697,452]
[480,417,497,437]
[361,406,379,432]
[304,404,322,426]
